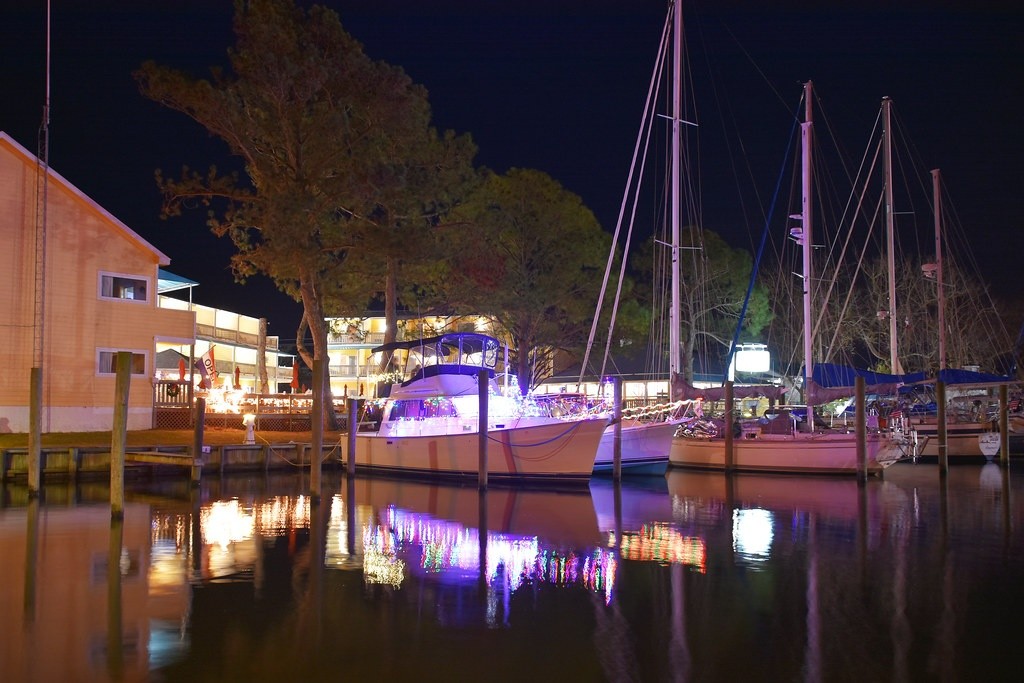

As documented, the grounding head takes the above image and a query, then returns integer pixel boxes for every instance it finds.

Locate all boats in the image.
[339,327,612,490]
[523,394,699,474]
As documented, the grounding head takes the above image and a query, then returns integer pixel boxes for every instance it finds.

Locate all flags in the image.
[193,347,221,390]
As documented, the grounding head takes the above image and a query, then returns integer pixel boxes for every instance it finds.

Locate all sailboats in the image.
[704,73,1003,463]
[570,1,912,479]
[920,160,1024,460]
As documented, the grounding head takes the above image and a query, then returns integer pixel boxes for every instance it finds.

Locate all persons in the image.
[1006,390,1024,414]
[972,398,987,424]
